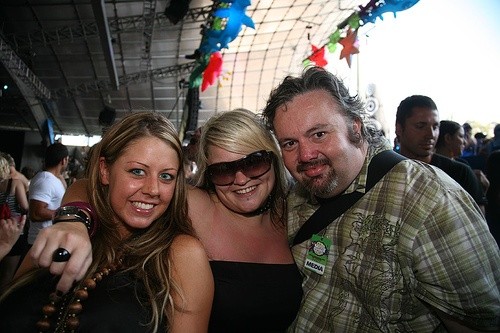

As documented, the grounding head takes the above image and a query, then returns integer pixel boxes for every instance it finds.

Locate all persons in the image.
[0,66,500,333]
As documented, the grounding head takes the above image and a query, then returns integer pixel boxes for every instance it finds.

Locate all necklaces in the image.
[37,254,125,333]
[248,193,272,214]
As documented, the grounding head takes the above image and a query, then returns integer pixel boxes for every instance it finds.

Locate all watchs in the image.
[55,207,91,234]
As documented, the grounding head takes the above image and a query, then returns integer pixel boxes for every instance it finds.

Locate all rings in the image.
[53,247,71,262]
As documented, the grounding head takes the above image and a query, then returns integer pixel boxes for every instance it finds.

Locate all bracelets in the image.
[56,201,97,236]
[52,217,88,224]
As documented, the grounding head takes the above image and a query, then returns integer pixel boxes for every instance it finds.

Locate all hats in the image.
[474,132,487,139]
[463,122,471,130]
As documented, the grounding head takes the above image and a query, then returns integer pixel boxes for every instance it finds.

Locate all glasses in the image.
[206,150,274,186]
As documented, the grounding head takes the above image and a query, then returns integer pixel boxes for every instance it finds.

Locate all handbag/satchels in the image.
[0,200,12,220]
